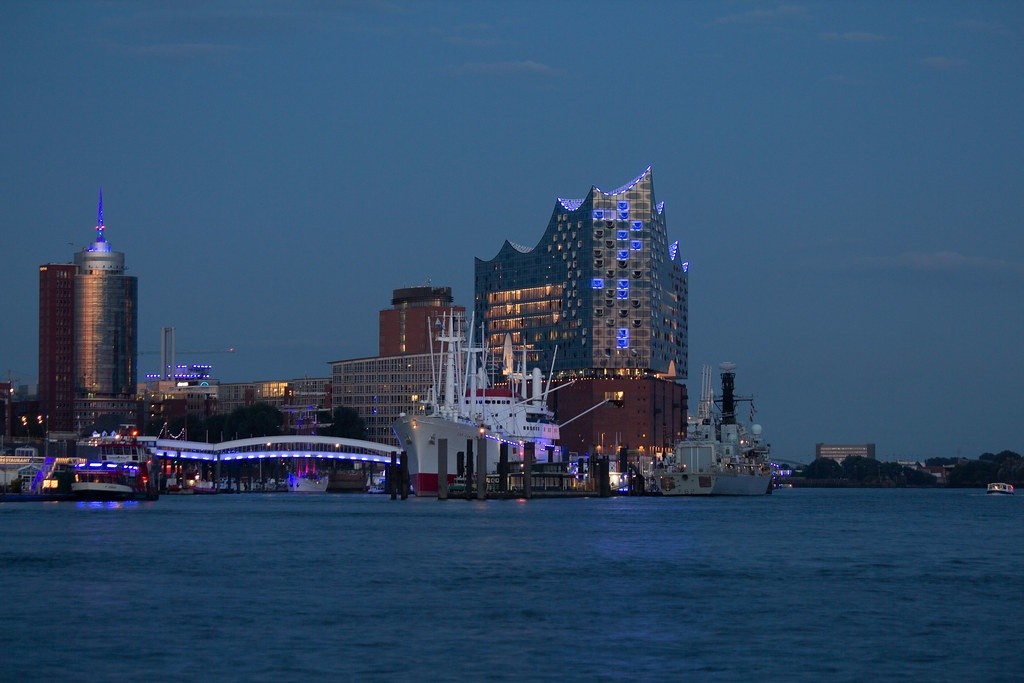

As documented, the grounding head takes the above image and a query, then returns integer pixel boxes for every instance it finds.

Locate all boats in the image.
[70,429,160,504]
[986,482,1015,496]
[653,362,776,497]
